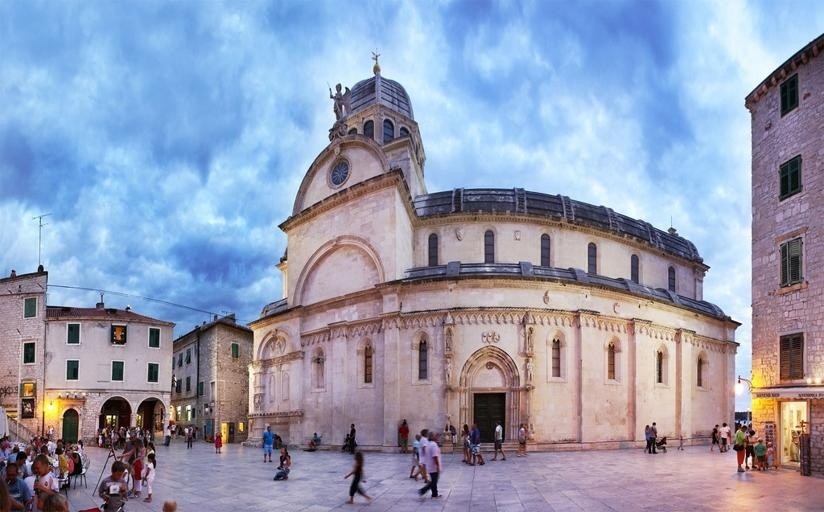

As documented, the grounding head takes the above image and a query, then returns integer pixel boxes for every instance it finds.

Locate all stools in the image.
[55,477,67,497]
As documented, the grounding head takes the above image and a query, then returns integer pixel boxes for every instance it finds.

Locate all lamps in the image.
[732,375,753,393]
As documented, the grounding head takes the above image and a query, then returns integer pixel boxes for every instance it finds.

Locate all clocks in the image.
[326,157,351,189]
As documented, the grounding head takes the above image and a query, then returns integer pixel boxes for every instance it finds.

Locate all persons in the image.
[330,83,342,120]
[263,426,291,482]
[349,423,356,454]
[342,434,350,450]
[643,422,667,454]
[517,423,526,457]
[344,452,375,504]
[214,431,222,454]
[309,432,321,450]
[710,419,778,473]
[490,420,506,461]
[0,423,194,512]
[398,418,485,500]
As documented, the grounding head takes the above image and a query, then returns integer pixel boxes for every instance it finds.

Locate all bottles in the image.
[33,475,40,495]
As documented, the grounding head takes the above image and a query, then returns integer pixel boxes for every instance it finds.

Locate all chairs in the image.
[67,452,89,489]
